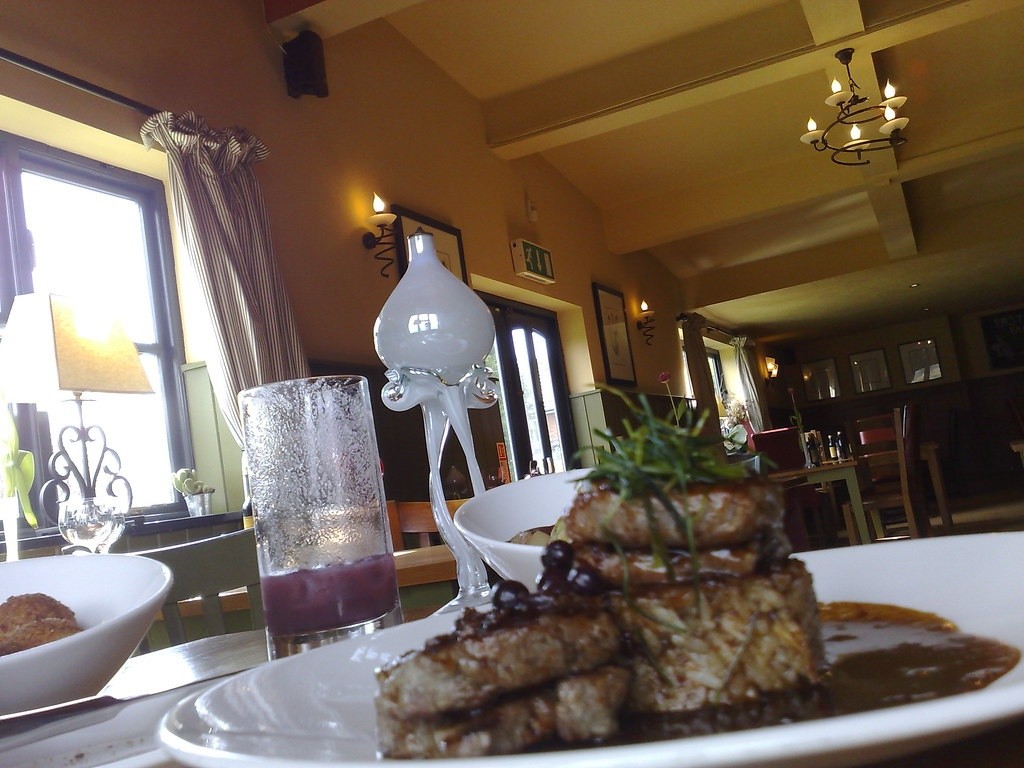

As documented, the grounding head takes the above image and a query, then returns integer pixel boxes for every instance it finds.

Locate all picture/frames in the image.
[592,282,637,386]
[898,336,943,384]
[980,309,1024,369]
[848,348,893,394]
[799,357,842,403]
[391,203,466,283]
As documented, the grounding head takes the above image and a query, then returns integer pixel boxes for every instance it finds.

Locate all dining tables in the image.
[0,604,436,768]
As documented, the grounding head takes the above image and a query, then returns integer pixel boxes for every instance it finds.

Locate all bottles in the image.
[808,436,821,468]
[837,431,849,459]
[827,435,838,461]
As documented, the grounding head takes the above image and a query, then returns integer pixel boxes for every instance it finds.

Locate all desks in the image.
[871,441,952,530]
[771,461,871,545]
[153,543,455,623]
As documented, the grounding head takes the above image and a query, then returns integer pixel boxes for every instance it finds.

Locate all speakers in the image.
[282,30,328,99]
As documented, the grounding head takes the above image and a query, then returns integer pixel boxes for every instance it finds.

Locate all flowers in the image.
[658,370,681,426]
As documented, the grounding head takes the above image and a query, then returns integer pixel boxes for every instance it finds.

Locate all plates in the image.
[157,529,1024,768]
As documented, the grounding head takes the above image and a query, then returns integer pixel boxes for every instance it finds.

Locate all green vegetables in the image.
[570,381,780,683]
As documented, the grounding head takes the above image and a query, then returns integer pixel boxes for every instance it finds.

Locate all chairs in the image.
[841,401,924,546]
[925,406,964,498]
[385,499,470,552]
[752,426,823,538]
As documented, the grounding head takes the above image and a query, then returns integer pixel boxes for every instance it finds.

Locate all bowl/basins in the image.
[0,554,176,720]
[454,468,597,595]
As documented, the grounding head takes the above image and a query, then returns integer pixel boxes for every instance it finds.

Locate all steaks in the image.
[374,476,831,758]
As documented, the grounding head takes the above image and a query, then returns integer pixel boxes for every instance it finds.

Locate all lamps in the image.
[363,189,396,278]
[716,395,731,418]
[800,47,909,166]
[635,298,655,345]
[764,357,778,384]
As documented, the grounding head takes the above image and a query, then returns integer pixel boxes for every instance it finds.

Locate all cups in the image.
[236,374,405,662]
